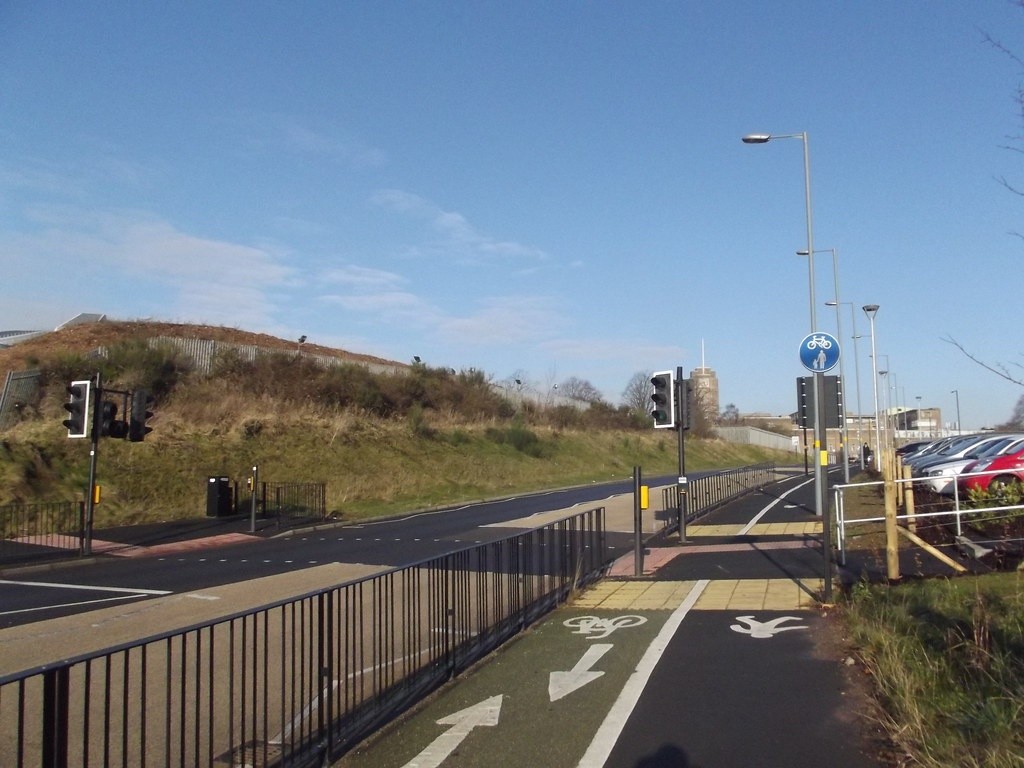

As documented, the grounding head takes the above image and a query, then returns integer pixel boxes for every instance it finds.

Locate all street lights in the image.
[795,248,847,483]
[824,301,865,471]
[868,354,896,450]
[915,397,922,443]
[952,390,961,437]
[891,386,907,445]
[864,303,882,471]
[742,131,824,516]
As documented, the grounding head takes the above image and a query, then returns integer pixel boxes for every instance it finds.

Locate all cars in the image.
[893,431,1024,507]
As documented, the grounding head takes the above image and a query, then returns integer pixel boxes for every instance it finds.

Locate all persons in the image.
[862,441,871,468]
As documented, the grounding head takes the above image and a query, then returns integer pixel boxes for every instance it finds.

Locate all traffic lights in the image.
[63,380,90,438]
[131,391,155,442]
[651,370,675,428]
[100,399,129,440]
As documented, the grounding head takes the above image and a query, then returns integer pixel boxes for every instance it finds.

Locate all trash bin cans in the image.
[827,452,838,464]
[206,476,229,517]
